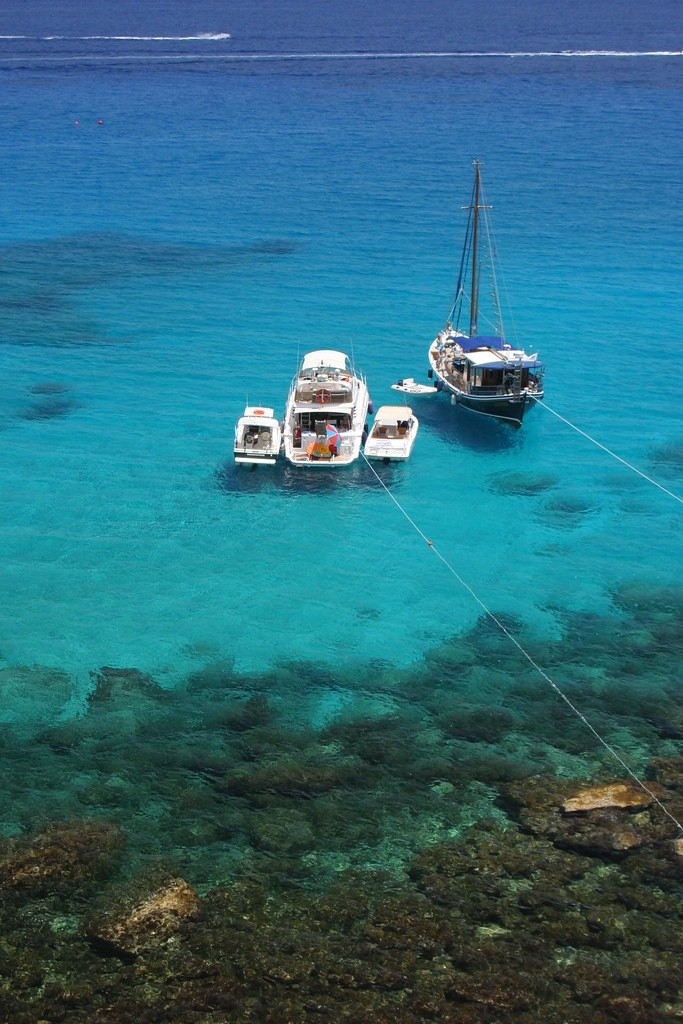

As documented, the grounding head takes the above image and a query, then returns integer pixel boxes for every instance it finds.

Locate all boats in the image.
[390,378,438,396]
[281,350,369,467]
[232,406,281,465]
[363,406,419,462]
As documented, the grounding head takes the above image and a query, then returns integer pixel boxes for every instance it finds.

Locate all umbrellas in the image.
[307,442,317,453]
[326,424,342,447]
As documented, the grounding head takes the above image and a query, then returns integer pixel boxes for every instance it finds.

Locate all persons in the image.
[437,351,451,364]
[437,336,443,347]
[244,427,258,443]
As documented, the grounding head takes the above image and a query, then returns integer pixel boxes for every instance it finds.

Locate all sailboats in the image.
[426,158,543,427]
